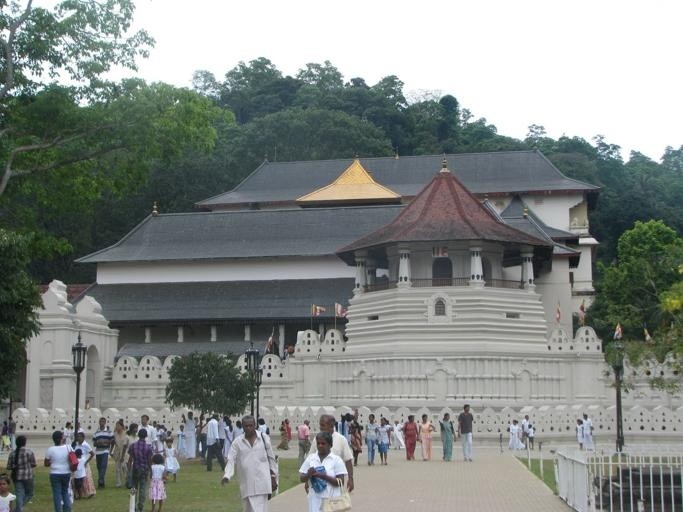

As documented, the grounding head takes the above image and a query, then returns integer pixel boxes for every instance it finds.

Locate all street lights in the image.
[244,349,259,429]
[254,367,261,428]
[70,331,86,450]
[610,346,623,453]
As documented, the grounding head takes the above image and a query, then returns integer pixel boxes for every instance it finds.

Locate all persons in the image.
[582,413,594,452]
[520,413,529,449]
[0,412,357,512]
[356,403,474,466]
[507,419,525,450]
[525,423,535,450]
[574,418,583,450]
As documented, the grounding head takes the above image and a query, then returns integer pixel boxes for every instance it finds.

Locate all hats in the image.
[53,431,64,440]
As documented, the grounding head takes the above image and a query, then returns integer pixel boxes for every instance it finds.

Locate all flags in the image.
[613,322,623,342]
[555,301,561,325]
[335,302,348,318]
[264,333,272,354]
[578,299,587,326]
[312,304,325,318]
[643,328,651,342]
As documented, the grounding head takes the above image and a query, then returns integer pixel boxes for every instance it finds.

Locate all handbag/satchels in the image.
[320,478,352,512]
[68,444,79,471]
[268,469,279,499]
